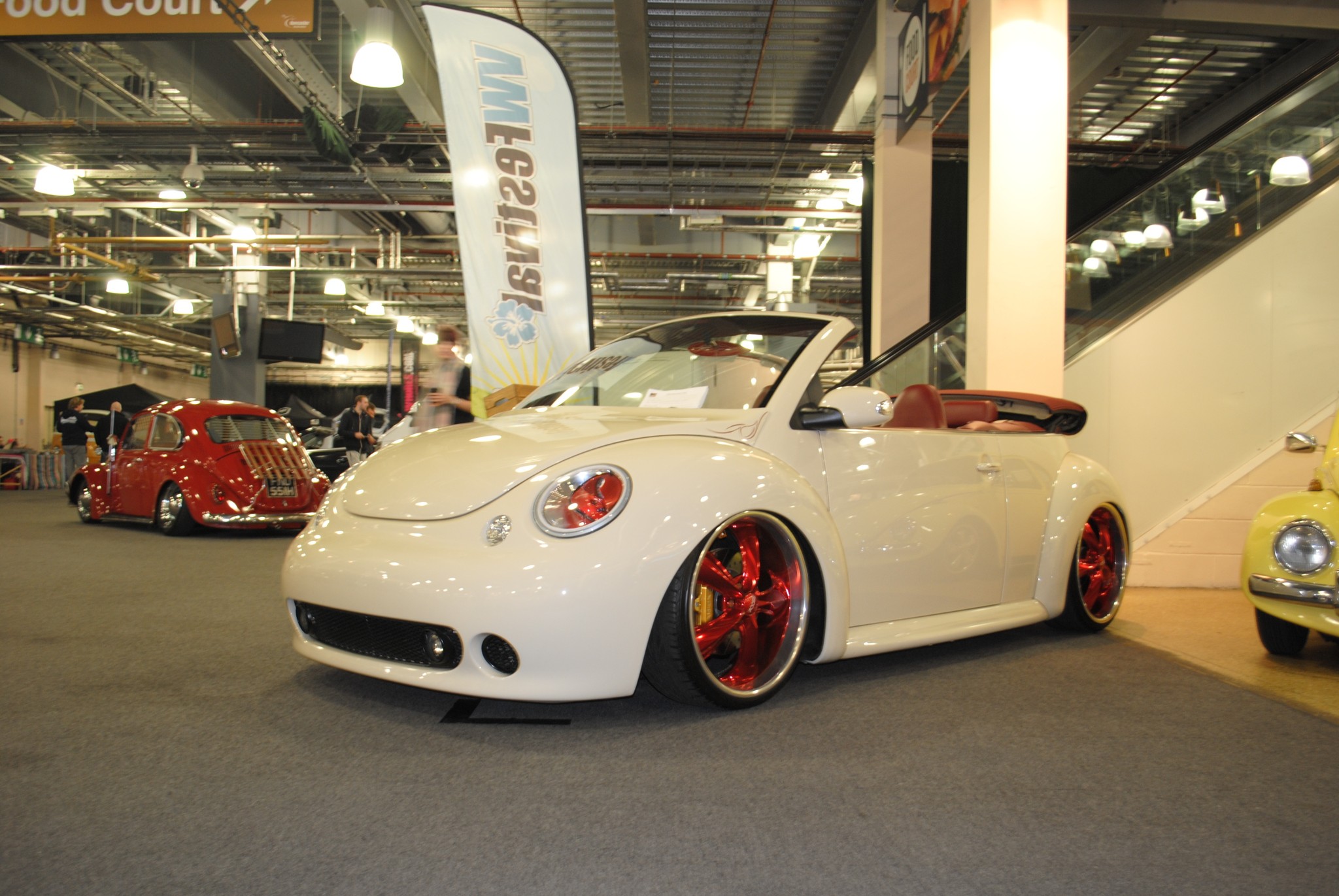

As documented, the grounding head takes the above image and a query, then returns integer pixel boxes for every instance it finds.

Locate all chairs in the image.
[880,384,947,429]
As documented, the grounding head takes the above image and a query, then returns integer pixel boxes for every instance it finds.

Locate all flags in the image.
[420,1,595,419]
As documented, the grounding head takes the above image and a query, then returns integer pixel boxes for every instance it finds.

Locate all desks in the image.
[0,454,66,490]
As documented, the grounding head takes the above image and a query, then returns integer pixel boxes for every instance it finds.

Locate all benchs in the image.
[944,400,1045,431]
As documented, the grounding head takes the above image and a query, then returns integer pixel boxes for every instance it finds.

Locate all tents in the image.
[47,384,180,451]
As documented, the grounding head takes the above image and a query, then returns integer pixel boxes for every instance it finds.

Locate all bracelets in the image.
[458,398,461,404]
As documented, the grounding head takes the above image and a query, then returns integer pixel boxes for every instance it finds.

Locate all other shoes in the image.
[64,481,70,495]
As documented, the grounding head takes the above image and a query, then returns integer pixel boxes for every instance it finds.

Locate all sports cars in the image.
[278,311,1129,712]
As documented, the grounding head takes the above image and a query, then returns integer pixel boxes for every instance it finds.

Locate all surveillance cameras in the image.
[181,164,205,189]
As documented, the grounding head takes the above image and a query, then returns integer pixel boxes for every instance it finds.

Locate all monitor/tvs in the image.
[213,312,242,359]
[258,318,325,364]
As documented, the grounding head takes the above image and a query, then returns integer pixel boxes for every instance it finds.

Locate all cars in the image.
[1240,408,1339,661]
[63,397,333,535]
[294,426,356,481]
[332,406,393,443]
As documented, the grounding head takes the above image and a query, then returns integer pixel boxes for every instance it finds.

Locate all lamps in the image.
[350,8,404,88]
[106,280,129,294]
[50,345,61,359]
[173,300,194,315]
[396,316,414,333]
[365,302,385,316]
[182,147,204,189]
[34,166,75,196]
[324,279,347,295]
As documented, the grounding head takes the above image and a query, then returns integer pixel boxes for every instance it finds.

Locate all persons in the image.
[418,324,474,431]
[56,397,95,495]
[337,395,376,468]
[94,401,133,463]
[0,436,17,450]
